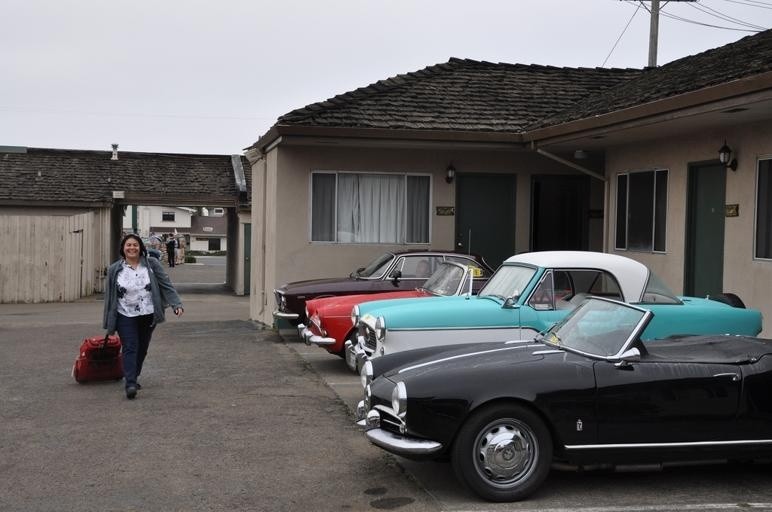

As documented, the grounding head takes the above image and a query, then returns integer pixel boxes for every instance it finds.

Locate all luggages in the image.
[71,325,126,386]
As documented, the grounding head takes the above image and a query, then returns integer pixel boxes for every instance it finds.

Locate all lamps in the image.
[718,139,737,171]
[445,164,456,184]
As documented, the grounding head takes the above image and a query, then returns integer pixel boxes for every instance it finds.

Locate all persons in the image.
[166,233,176,268]
[102,232,185,400]
[174,234,180,265]
[178,234,185,264]
[147,236,162,261]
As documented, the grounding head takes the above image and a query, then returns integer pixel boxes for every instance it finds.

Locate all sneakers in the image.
[126,381,142,398]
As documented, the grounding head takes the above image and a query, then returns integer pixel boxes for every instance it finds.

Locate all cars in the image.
[345,250,763,375]
[272,250,497,330]
[297,260,572,358]
[355,294,772,503]
